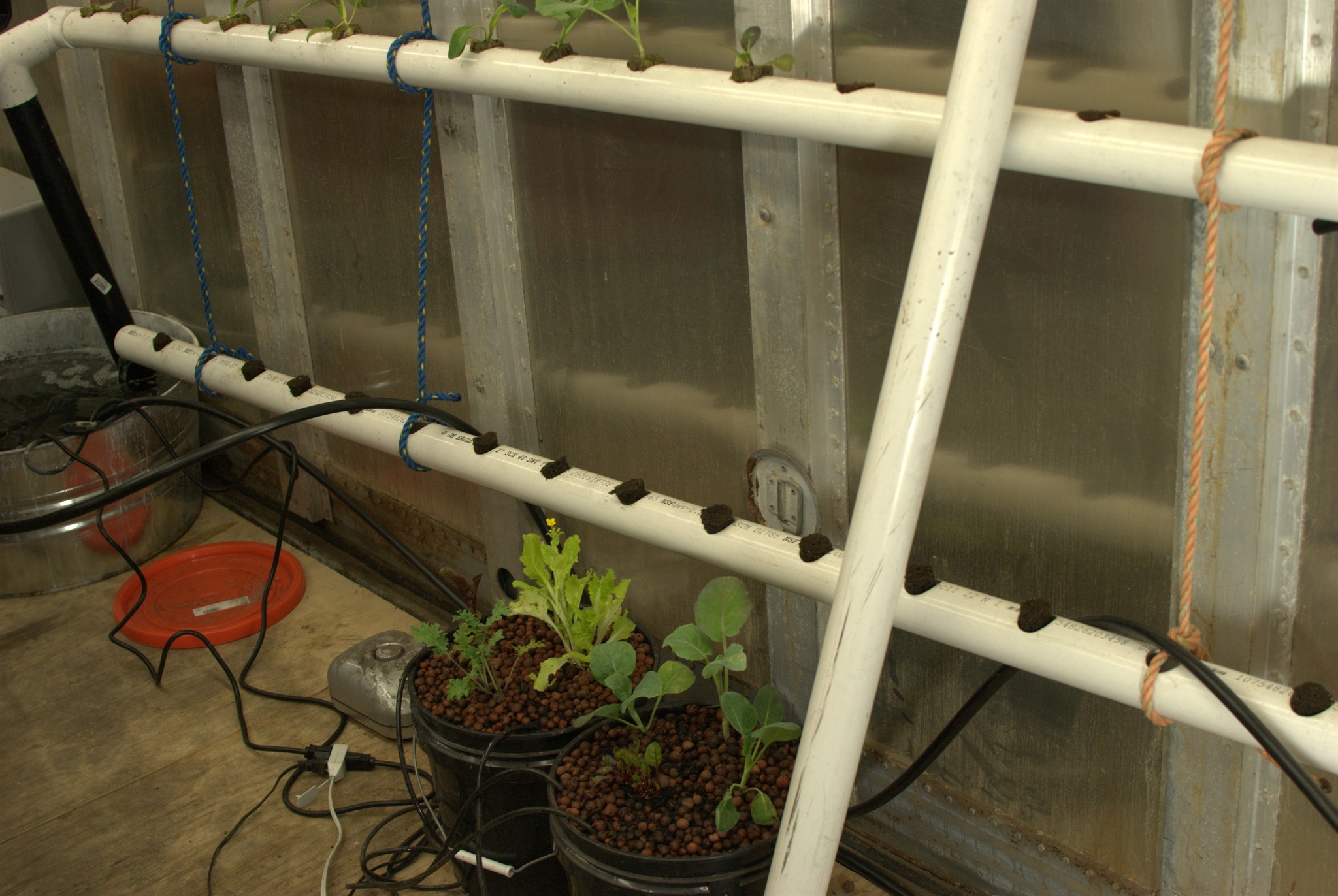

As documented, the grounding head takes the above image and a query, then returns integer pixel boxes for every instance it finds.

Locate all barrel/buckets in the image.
[0,306,204,597]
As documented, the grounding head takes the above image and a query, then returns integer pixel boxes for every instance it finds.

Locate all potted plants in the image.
[391,512,798,896]
